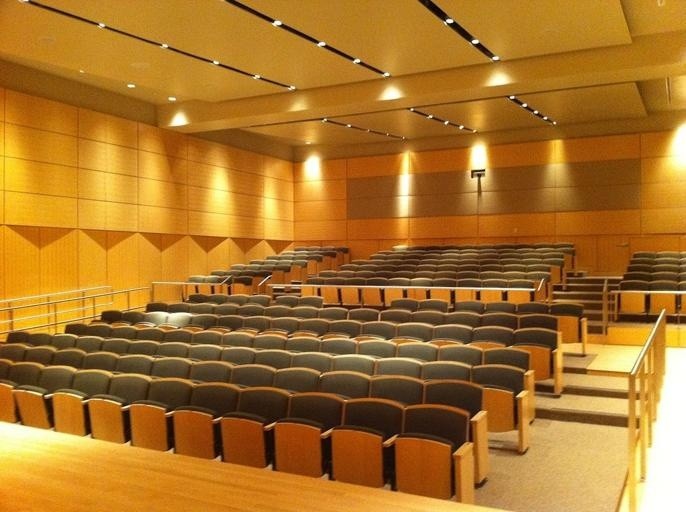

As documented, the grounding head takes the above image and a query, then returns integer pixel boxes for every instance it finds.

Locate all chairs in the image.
[1,287,591,501]
[610,243,684,326]
[301,241,579,303]
[183,242,352,294]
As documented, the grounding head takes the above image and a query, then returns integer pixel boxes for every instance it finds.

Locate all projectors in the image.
[473,172,485,177]
